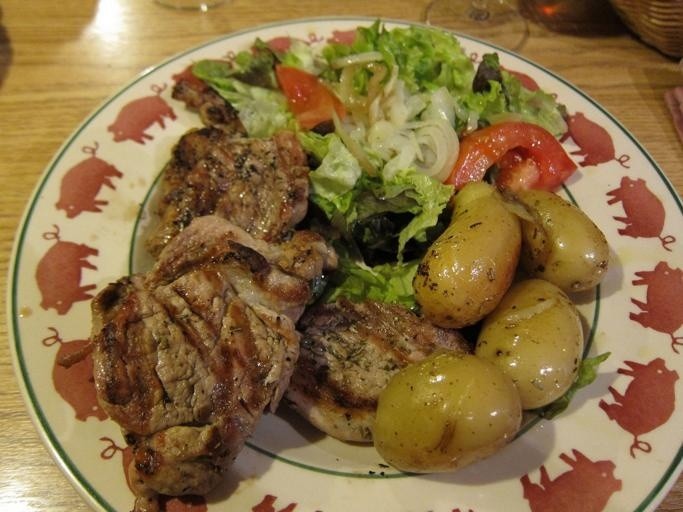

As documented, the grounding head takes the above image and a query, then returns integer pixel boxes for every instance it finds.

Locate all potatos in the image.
[415,183,521,329]
[476,280,584,411]
[512,191,609,293]
[375,350,522,473]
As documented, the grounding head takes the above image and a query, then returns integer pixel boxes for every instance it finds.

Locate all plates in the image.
[8,14,683,512]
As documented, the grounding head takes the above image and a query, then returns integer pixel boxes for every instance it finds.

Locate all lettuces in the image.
[191,19,567,314]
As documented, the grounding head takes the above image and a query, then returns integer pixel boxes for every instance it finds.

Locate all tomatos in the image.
[446,121,577,191]
